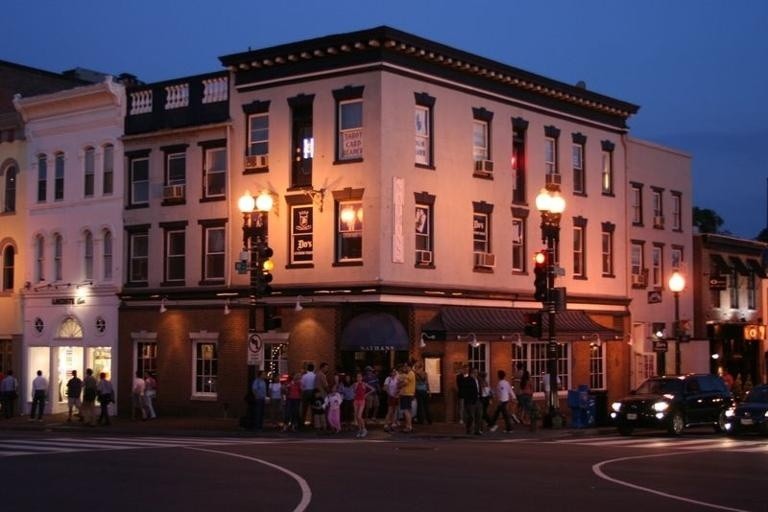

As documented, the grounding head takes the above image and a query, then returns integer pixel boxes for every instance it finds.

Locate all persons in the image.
[252,358,431,438]
[457,364,561,436]
[720,368,753,403]
[0,369,157,427]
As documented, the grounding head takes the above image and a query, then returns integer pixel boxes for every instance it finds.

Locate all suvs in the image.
[609,372,734,438]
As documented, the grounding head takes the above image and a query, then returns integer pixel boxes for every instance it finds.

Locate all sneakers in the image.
[384,424,413,433]
[356,430,361,438]
[360,429,367,437]
[490,413,520,434]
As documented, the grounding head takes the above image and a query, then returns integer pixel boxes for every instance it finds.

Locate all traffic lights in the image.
[258,244,273,297]
[532,250,547,303]
[523,310,539,336]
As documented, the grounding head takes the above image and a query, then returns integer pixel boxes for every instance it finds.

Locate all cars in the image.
[723,383,768,435]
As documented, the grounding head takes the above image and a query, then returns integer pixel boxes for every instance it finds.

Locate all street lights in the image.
[530,189,566,430]
[235,189,273,427]
[664,272,688,374]
